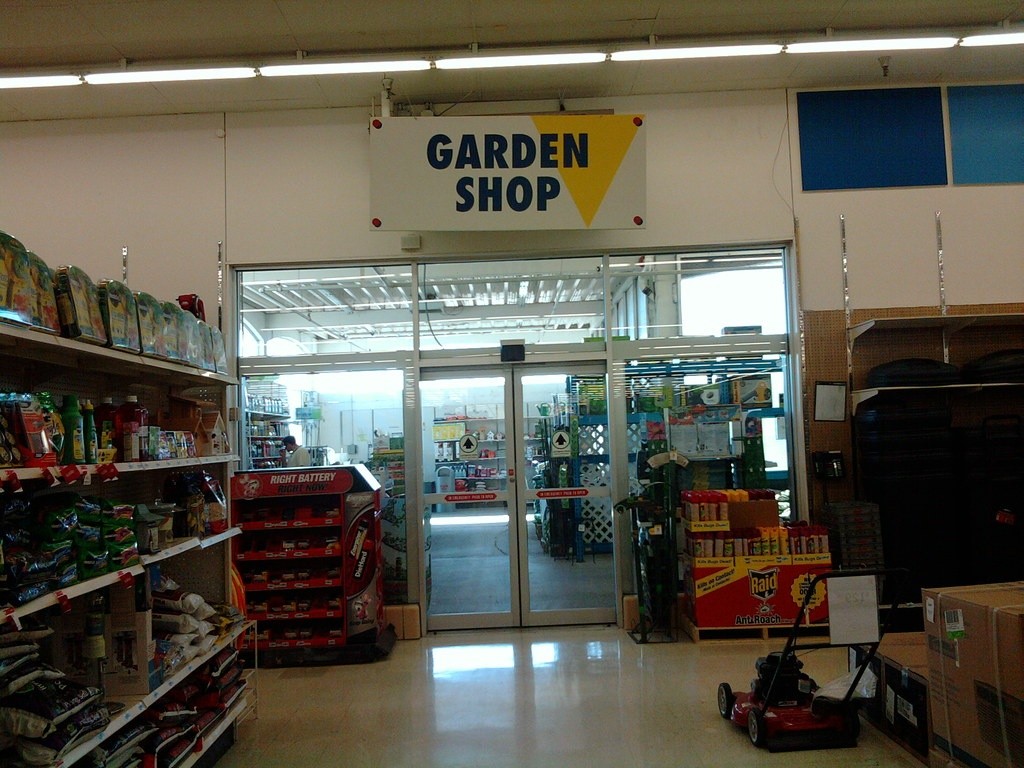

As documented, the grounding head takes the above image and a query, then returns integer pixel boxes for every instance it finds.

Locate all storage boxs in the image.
[578,373,773,416]
[373,437,405,496]
[848,630,929,766]
[928,748,962,767]
[920,581,1024,767]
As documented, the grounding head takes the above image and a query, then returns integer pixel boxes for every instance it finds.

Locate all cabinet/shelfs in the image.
[1,322,258,767]
[229,464,386,648]
[245,409,289,468]
[847,312,1024,416]
[435,417,546,503]
